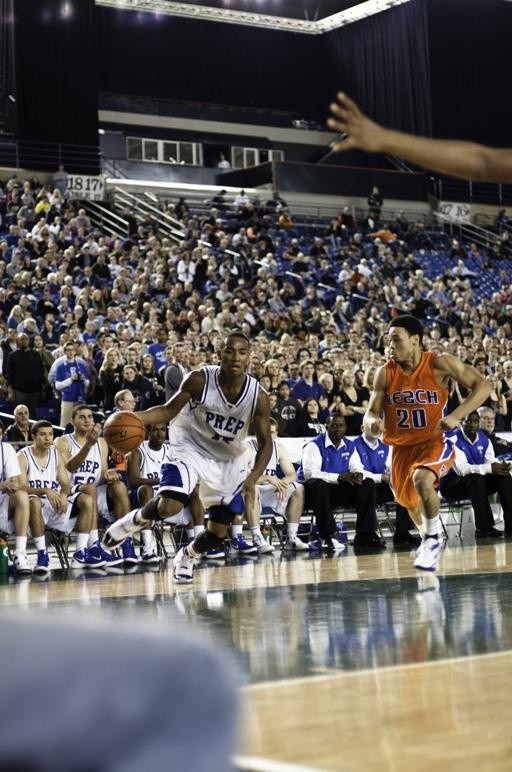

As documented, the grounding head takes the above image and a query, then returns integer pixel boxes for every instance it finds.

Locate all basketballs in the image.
[103,411,145,451]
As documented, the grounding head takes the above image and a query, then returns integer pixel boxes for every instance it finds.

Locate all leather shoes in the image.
[475,527,503,537]
[393,531,421,545]
[317,534,334,550]
[353,532,388,547]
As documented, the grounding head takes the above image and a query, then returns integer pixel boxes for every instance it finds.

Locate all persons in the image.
[325,87,512,192]
[217,154,231,169]
[1,166,512,586]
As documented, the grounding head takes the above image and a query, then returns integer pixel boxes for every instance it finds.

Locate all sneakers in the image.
[227,533,257,555]
[100,507,151,551]
[172,545,195,585]
[252,534,275,555]
[70,537,164,569]
[187,535,225,565]
[34,549,50,574]
[413,526,444,571]
[281,537,309,551]
[11,552,33,574]
